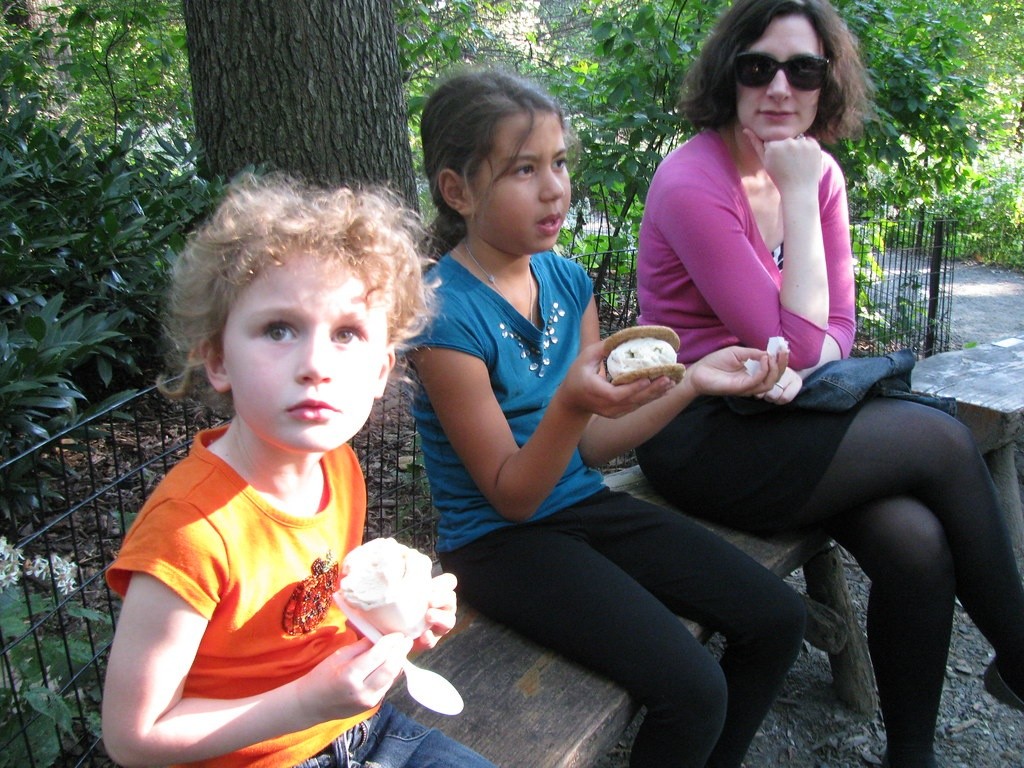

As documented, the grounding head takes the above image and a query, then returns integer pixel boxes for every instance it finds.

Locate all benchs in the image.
[909,332,1024,590]
[378,461,881,768]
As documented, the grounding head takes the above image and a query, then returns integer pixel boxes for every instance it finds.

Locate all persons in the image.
[397,68,812,768]
[628,1,1024,768]
[96,158,503,768]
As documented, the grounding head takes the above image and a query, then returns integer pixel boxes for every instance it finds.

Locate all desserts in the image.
[339,536,432,640]
[601,325,684,386]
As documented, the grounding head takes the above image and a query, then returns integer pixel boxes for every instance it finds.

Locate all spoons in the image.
[329,589,464,716]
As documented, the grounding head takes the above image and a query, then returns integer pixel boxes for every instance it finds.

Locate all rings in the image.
[774,382,785,390]
[794,133,807,141]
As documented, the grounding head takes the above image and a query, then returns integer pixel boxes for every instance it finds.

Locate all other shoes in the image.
[984,662,1023,714]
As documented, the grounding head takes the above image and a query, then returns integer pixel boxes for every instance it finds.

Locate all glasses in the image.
[734,52,830,91]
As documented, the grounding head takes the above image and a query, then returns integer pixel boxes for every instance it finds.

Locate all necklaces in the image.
[461,236,534,328]
